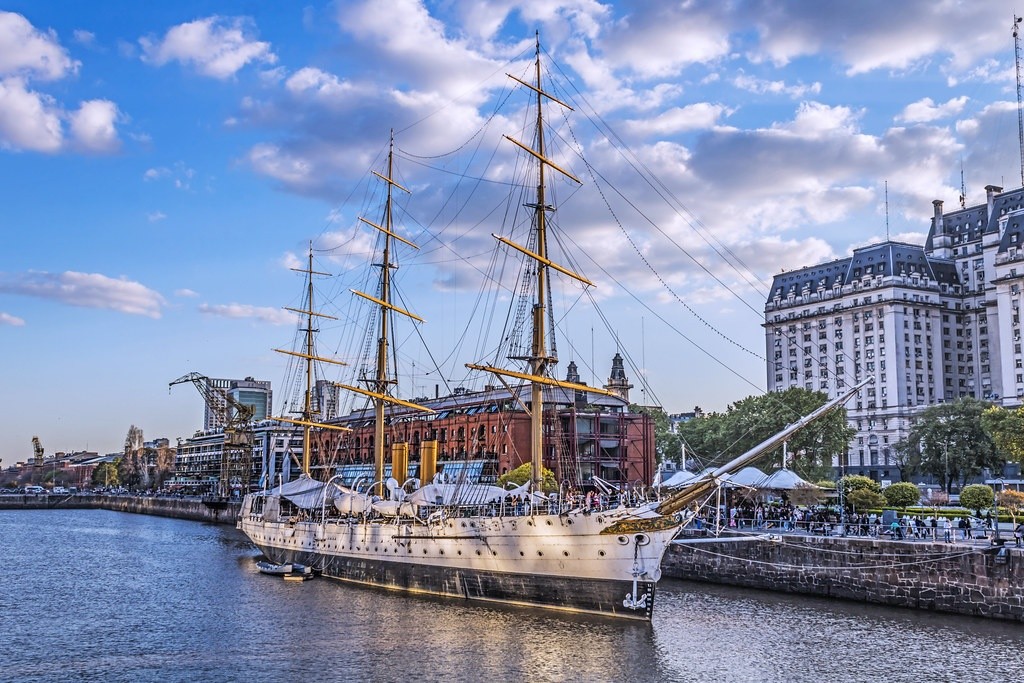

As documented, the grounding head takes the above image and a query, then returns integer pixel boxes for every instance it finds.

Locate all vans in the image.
[25,485,77,494]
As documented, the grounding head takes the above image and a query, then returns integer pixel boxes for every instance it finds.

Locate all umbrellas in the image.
[658,466,819,505]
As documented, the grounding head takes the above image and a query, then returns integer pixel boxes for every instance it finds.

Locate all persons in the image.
[1,485,1023,553]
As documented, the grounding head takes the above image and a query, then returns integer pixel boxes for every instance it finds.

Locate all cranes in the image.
[168,371,257,499]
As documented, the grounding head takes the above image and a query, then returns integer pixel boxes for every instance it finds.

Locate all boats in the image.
[287,562,311,573]
[255,561,292,574]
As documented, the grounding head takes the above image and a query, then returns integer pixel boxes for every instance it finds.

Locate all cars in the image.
[0,489,25,494]
[136,491,184,499]
[90,487,128,495]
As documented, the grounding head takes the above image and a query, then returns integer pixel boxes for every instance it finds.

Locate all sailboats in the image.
[236,29,873,625]
